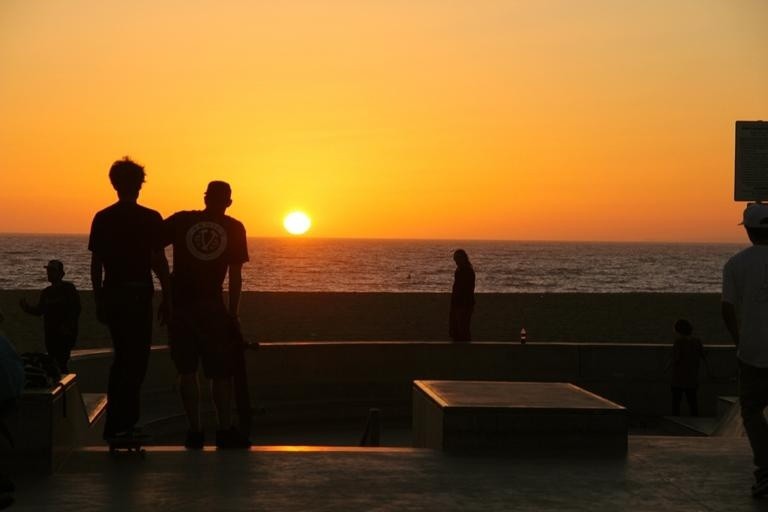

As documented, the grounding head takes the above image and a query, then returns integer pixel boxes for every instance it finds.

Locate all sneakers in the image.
[751,466,768,497]
[102,424,144,441]
[215,423,252,449]
[184,427,204,450]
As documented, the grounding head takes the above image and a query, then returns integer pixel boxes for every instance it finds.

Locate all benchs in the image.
[412,379,629,456]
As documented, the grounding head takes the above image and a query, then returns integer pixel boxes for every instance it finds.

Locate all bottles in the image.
[520,327,527,344]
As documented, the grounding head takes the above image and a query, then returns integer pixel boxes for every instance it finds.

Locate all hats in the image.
[738,202,768,229]
[43,259,65,270]
[204,182,232,195]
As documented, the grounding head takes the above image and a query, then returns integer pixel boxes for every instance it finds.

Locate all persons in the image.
[669,317,704,416]
[147,180,252,451]
[19,258,80,364]
[448,246,475,341]
[87,157,163,445]
[721,201,768,499]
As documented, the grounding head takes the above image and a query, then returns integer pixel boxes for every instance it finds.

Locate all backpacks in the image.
[19,351,62,390]
